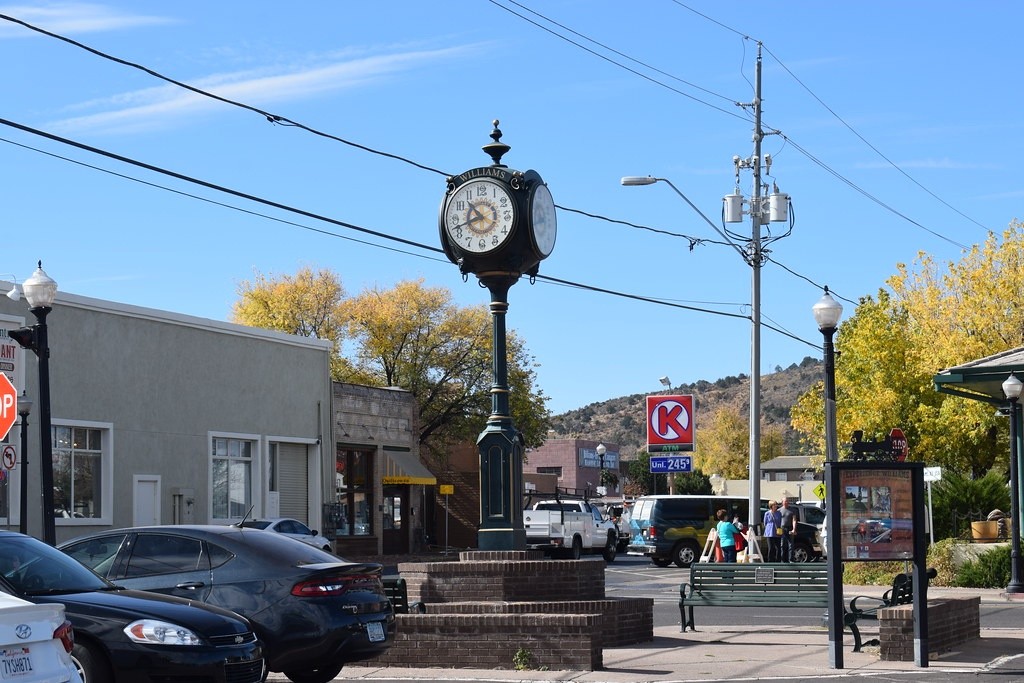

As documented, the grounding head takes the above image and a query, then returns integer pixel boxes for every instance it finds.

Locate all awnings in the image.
[343,455,368,485]
[383,449,437,485]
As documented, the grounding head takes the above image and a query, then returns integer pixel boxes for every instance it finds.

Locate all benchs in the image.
[844,568,937,653]
[382,579,427,614]
[679,563,845,633]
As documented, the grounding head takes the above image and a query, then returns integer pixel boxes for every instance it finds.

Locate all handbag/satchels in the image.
[777,528,782,536]
[734,533,744,551]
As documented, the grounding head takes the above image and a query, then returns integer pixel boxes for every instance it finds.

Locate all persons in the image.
[777,497,797,563]
[716,509,743,580]
[763,500,785,562]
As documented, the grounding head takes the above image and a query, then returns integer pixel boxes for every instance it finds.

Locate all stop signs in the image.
[889,428,908,462]
[0,372,18,441]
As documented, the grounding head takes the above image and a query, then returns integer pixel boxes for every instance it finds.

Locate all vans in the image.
[627,495,823,568]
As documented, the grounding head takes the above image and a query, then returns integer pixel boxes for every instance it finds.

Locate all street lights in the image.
[16,390,33,533]
[619,174,761,565]
[812,286,845,669]
[8,258,60,546]
[1001,370,1024,594]
[596,441,607,487]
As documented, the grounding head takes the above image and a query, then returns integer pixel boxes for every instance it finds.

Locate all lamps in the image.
[660,376,671,395]
[0,273,20,302]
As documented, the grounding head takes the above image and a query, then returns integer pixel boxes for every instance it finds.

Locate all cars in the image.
[852,519,892,544]
[0,590,84,683]
[0,528,269,683]
[6,525,397,683]
[233,517,332,552]
[777,503,825,529]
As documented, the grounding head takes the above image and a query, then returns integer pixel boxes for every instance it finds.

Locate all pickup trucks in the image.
[523,486,620,564]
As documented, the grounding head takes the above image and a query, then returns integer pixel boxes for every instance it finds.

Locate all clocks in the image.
[445,167,521,283]
[524,169,558,285]
